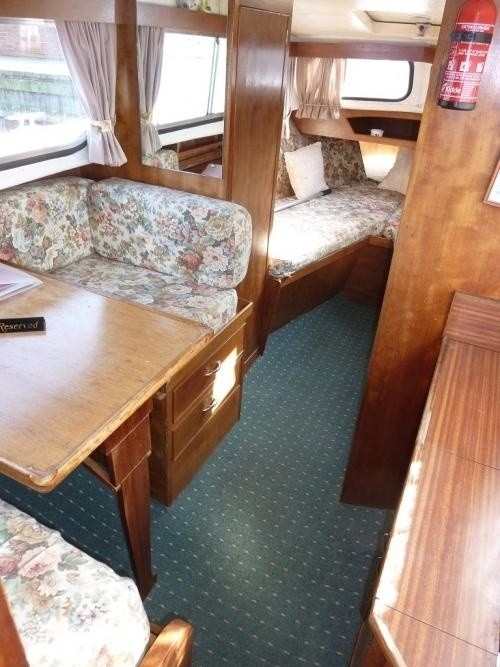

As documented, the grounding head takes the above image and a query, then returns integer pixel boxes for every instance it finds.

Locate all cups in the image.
[371,129,384,137]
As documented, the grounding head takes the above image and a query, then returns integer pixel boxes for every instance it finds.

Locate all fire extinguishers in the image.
[437,1,497,111]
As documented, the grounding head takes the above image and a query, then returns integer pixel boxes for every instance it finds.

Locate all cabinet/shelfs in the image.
[151,323,242,506]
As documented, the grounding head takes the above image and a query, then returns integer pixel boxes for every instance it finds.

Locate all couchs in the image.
[0,178,253,393]
[143,128,407,354]
[0,499,194,667]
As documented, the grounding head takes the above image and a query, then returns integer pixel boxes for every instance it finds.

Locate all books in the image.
[0,264,44,304]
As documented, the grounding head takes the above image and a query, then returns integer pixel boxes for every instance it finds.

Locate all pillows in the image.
[377,144,414,196]
[284,142,330,200]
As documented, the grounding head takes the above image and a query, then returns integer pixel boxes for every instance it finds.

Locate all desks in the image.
[349,293,500,667]
[0,261,214,602]
